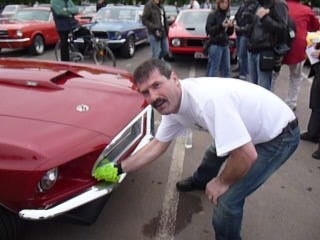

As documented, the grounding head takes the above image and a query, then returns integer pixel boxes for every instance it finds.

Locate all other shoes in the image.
[300,131,311,140]
[176,177,206,192]
[312,150,320,160]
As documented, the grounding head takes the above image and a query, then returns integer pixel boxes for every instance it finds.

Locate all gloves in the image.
[98,164,123,184]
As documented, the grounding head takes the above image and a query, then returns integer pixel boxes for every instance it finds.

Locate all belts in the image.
[279,117,298,136]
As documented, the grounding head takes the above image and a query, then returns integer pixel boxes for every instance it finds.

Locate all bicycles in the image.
[55,15,116,67]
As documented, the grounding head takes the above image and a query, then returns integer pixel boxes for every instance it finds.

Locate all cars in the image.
[163,5,181,23]
[306,17,320,66]
[77,5,110,21]
[1,5,28,17]
[0,57,156,224]
[167,9,237,64]
[0,6,90,56]
[70,4,150,57]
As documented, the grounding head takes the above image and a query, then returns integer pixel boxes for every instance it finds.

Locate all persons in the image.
[163,0,217,9]
[50,0,96,62]
[141,0,169,59]
[232,0,258,80]
[247,0,289,92]
[94,57,300,240]
[78,0,142,12]
[300,19,320,160]
[205,0,235,79]
[270,0,320,114]
[0,0,40,12]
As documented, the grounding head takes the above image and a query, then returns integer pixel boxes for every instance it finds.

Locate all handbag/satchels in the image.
[203,39,211,56]
[260,51,276,72]
